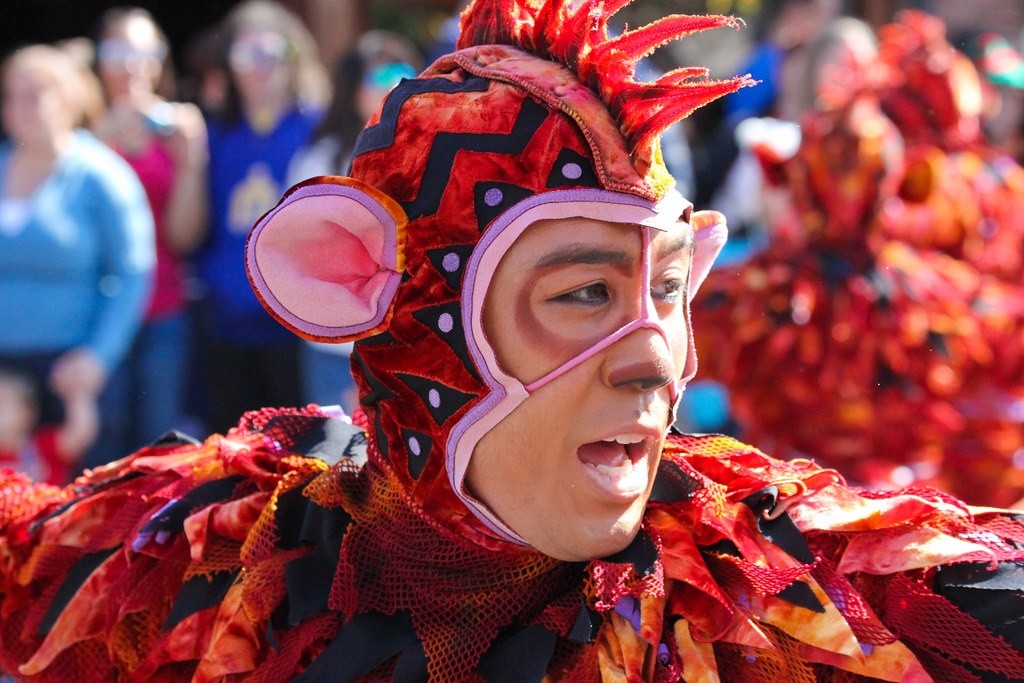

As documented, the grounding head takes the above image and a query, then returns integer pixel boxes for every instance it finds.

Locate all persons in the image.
[0,53,1024,683]
[0,1,463,489]
[622,1,1024,509]
